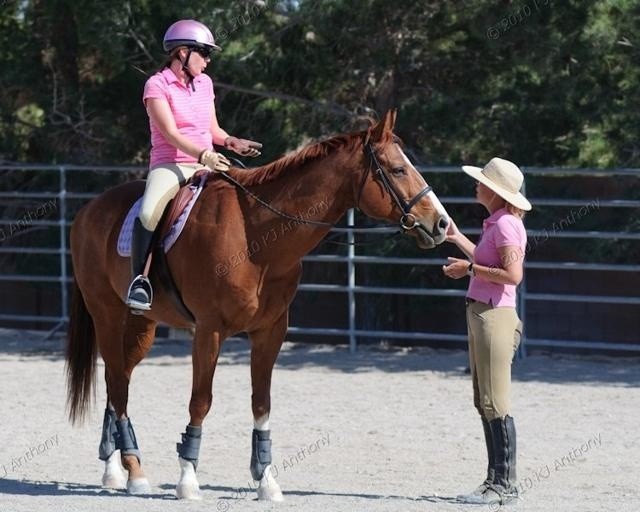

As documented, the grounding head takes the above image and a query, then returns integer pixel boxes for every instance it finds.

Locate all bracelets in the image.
[467,263,475,277]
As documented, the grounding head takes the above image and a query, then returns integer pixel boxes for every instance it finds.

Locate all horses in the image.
[62,107,453,503]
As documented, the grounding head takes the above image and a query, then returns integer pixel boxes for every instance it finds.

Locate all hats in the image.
[461,156,533,212]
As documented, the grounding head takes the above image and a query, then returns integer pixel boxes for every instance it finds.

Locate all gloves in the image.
[224,135,263,159]
[199,150,231,173]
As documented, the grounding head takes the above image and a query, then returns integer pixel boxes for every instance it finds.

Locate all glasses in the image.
[192,47,212,56]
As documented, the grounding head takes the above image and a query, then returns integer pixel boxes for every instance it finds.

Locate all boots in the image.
[455,414,518,505]
[130,216,155,315]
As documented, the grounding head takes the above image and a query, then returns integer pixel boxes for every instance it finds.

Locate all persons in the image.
[443,157,532,505]
[126,20,262,310]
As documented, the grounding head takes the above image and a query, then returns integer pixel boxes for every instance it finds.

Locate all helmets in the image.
[163,19,224,52]
